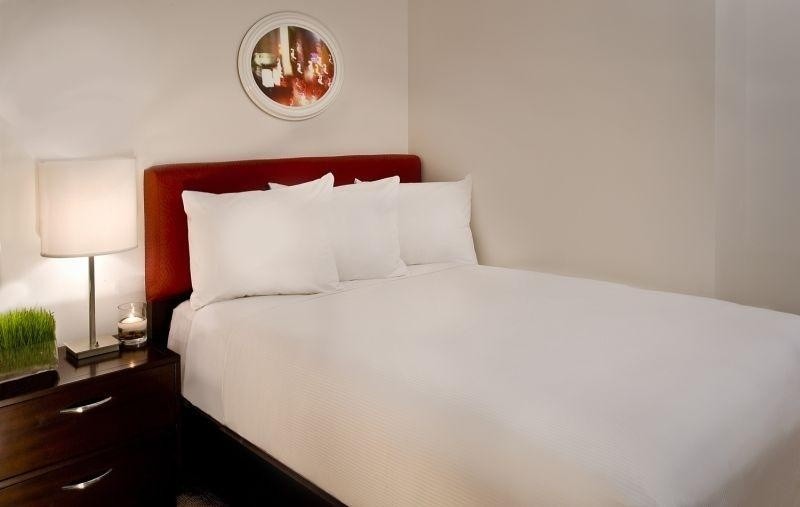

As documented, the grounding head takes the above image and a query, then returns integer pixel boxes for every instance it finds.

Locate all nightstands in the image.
[0,338,181,507]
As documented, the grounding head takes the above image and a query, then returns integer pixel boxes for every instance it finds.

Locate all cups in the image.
[116,303,148,352]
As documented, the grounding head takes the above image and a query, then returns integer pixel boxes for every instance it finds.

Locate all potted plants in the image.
[0,307,60,400]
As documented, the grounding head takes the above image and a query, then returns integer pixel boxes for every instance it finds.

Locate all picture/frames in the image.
[238,10,345,122]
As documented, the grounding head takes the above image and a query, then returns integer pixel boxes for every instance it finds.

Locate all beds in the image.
[143,154,800,507]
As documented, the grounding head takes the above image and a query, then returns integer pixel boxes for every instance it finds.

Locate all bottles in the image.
[254,52,276,88]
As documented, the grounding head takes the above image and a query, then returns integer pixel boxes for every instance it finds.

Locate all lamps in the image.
[39,155,139,361]
